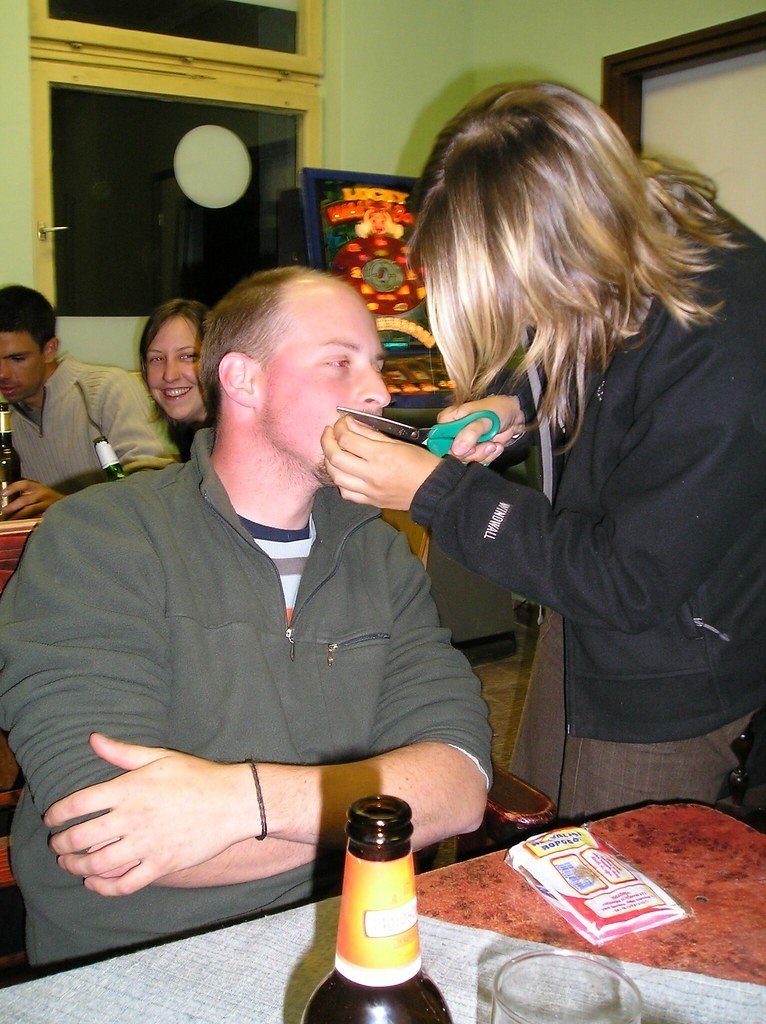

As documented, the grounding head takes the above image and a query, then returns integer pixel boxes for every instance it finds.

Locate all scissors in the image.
[337,402,499,471]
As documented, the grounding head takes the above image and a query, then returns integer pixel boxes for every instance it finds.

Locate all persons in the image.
[0,266,494,982]
[139,299,208,431]
[320,80,766,826]
[0,285,172,522]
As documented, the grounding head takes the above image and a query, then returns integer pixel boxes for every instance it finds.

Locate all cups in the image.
[491,953,643,1024]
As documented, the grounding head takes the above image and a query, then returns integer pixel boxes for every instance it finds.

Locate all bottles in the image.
[301,796,452,1024]
[0,402,22,522]
[94,437,131,481]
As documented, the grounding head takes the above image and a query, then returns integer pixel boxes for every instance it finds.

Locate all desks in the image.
[0,803,766,1024]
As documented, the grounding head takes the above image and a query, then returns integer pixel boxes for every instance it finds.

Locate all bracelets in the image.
[248,761,267,841]
